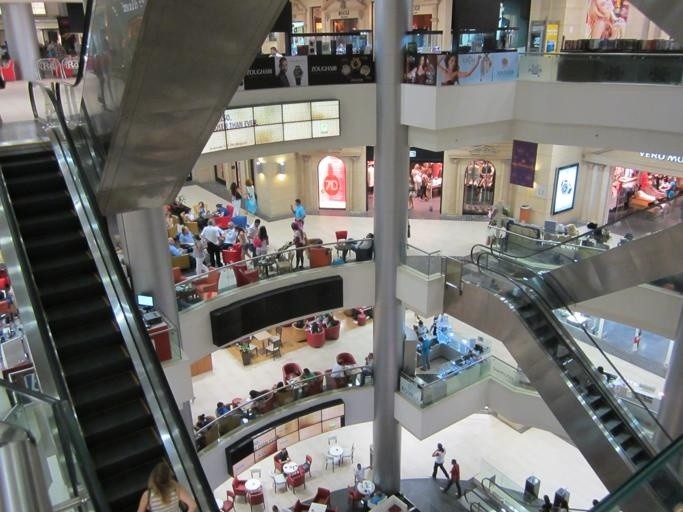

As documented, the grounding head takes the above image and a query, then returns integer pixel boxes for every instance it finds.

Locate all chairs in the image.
[164,206,349,299]
[193,305,369,447]
[221,435,377,512]
[489,208,630,259]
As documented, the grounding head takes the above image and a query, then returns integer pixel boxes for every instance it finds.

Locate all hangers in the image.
[612,163,664,218]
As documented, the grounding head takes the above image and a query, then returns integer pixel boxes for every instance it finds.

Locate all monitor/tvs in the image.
[137,295,154,308]
[544,221,556,233]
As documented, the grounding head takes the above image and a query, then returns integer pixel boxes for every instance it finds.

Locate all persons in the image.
[441,460,462,498]
[538,495,552,511]
[354,463,371,484]
[438,55,482,87]
[404,56,435,85]
[277,57,290,87]
[277,448,289,464]
[407,164,431,209]
[272,505,278,512]
[335,234,374,261]
[164,180,304,268]
[559,498,569,512]
[431,442,450,481]
[587,0,624,39]
[195,314,436,444]
[138,463,198,512]
[269,47,283,57]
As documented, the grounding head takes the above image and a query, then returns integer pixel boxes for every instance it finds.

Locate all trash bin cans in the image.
[519,205,531,225]
